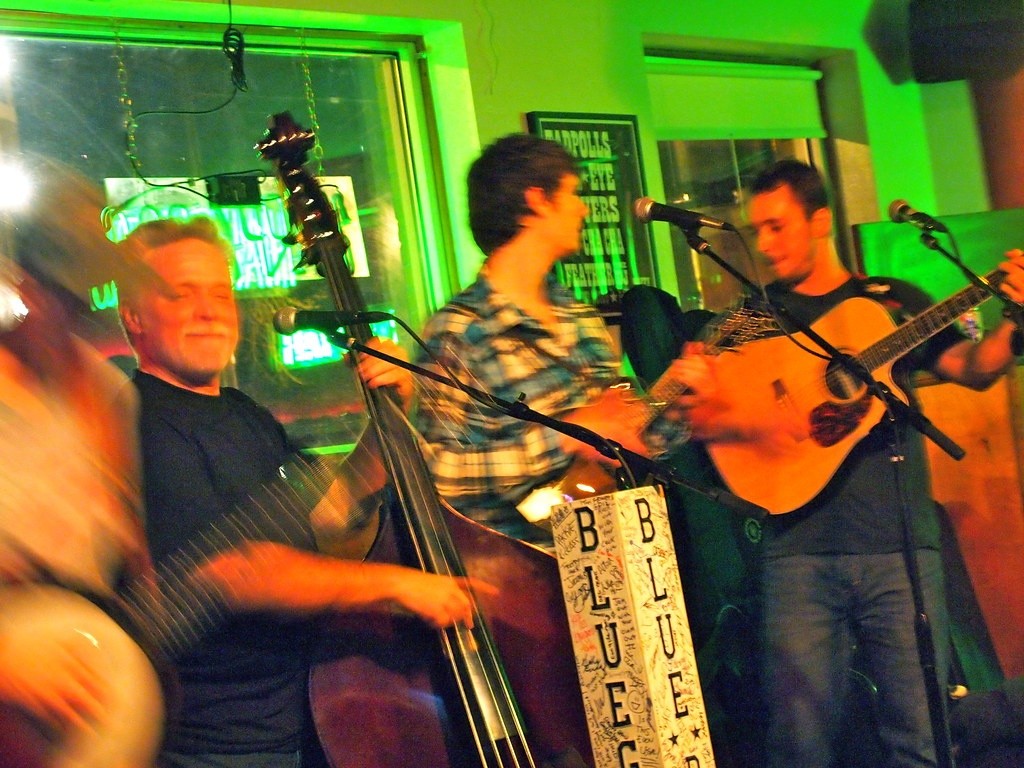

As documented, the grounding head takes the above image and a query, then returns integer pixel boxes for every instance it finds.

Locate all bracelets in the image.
[1003,303,1023,323]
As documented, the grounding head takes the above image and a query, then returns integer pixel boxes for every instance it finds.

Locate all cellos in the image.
[259,112,597,768]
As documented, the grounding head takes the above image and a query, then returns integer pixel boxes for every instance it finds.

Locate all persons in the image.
[420,132,744,767]
[96,218,500,766]
[680,161,1024,767]
[0,152,171,767]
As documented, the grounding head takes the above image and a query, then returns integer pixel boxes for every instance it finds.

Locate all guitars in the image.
[685,250,1012,518]
[512,305,773,538]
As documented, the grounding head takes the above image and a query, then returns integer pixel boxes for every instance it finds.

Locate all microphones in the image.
[633,196,735,232]
[889,199,949,232]
[273,308,392,335]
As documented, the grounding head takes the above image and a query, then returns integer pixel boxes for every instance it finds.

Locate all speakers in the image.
[910,0,1024,84]
